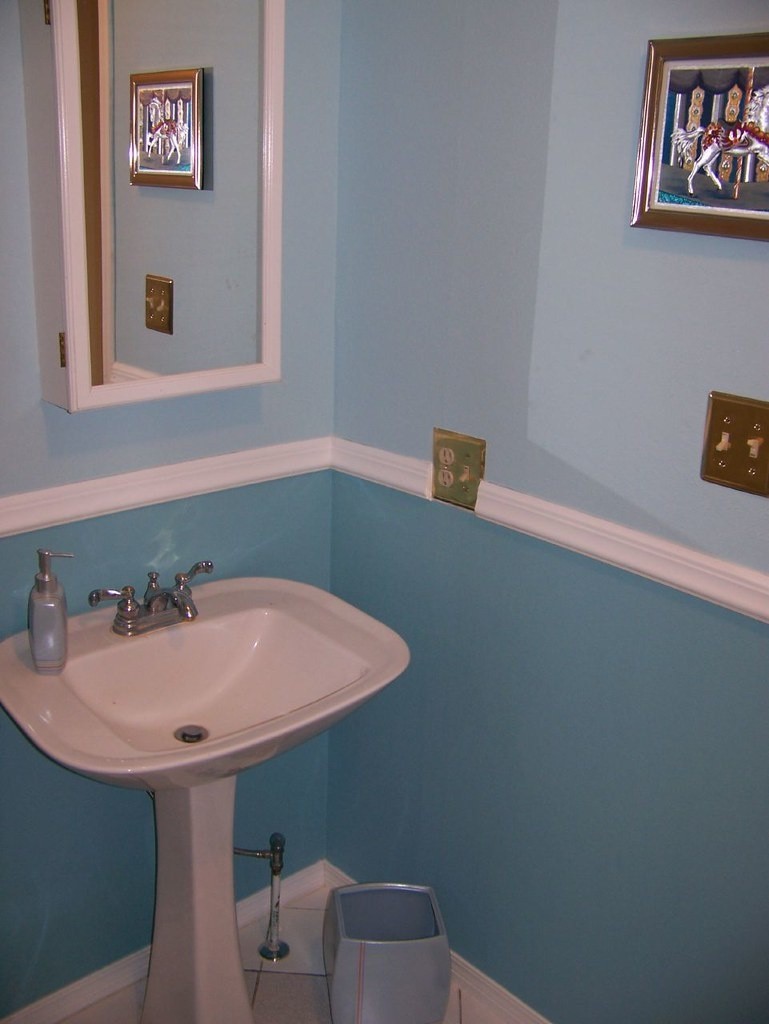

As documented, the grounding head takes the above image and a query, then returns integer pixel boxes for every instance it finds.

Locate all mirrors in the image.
[50,0,284,413]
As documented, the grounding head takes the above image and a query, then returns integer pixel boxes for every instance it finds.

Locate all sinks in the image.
[61,607,373,753]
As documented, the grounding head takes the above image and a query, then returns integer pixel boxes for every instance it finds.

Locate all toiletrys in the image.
[28,548,75,675]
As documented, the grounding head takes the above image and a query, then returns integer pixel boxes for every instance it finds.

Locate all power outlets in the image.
[431,428,484,509]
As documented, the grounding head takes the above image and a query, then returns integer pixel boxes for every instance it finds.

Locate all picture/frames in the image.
[129,67,204,190]
[630,32,769,242]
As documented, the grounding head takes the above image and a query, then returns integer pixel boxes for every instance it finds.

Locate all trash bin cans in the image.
[321,881,452,1024]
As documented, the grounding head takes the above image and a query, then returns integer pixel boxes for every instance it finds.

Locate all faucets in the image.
[88,560,214,636]
